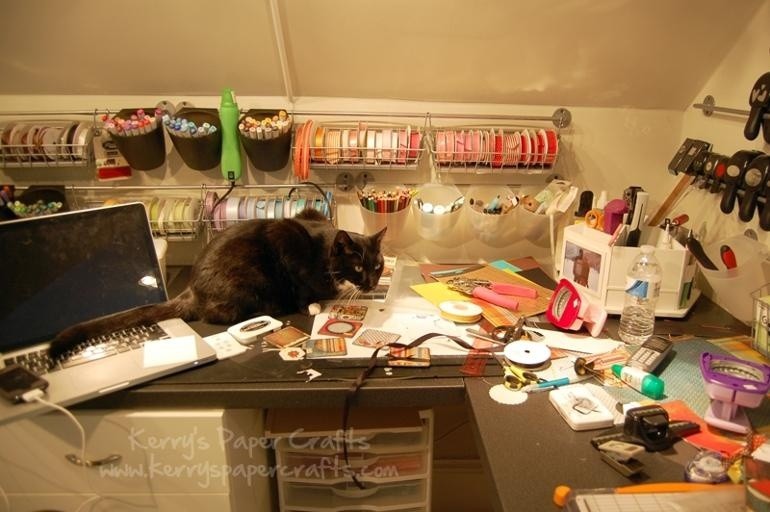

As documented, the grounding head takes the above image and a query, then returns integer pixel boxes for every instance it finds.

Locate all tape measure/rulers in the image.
[447,277,538,311]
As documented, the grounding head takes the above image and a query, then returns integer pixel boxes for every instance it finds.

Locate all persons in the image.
[572,248,590,287]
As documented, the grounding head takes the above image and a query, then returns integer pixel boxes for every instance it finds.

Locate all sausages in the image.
[46,217,390,362]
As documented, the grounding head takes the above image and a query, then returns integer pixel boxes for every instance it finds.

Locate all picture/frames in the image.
[68,264,769,512]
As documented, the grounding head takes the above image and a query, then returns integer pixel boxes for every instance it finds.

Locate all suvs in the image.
[235,110,292,171]
[165,109,222,171]
[109,107,162,171]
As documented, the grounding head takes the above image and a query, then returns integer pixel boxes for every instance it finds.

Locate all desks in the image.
[68,264,769,512]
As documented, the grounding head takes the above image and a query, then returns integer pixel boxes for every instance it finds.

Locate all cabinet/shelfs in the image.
[619,243,662,344]
[221,88,240,180]
[2,409,433,512]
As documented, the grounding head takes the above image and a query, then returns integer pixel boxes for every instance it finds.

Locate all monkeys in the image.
[554,486,573,506]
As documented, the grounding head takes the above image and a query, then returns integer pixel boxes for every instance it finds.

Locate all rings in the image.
[354,188,411,241]
[238,111,293,140]
[534,200,547,215]
[611,481,744,494]
[0,184,63,218]
[468,195,519,245]
[163,113,218,137]
[100,108,161,137]
[521,374,594,394]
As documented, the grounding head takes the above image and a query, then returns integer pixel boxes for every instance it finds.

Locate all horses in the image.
[626,335,674,373]
[0,364,49,405]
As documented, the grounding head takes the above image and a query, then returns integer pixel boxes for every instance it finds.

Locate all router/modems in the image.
[524,197,539,213]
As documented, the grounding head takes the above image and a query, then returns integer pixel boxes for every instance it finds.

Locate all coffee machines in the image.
[492,315,533,342]
[492,353,539,390]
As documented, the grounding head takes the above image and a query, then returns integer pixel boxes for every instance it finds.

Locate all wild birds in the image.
[0,201,217,426]
